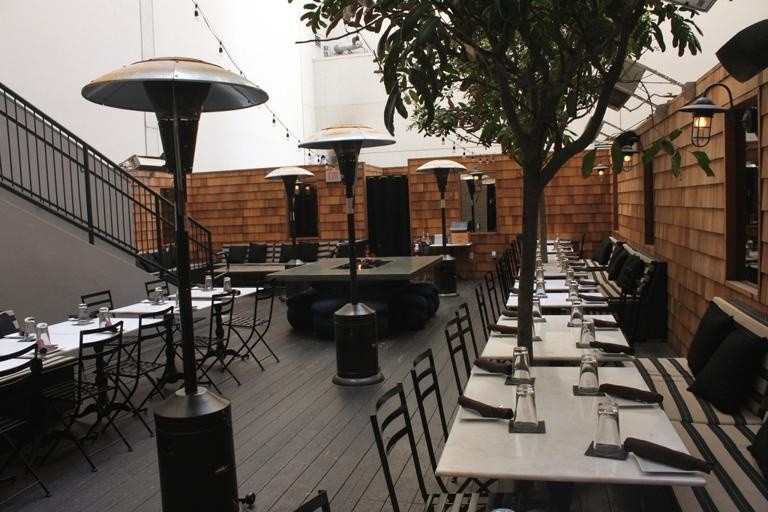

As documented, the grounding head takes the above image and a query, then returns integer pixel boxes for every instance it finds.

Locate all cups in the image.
[174,290,180,307]
[223,277,232,293]
[578,353,599,393]
[98,307,113,329]
[78,303,90,323]
[35,323,51,346]
[154,287,166,304]
[593,401,623,455]
[580,320,595,344]
[570,300,584,325]
[511,346,531,380]
[535,241,546,298]
[513,382,540,430]
[532,298,542,318]
[23,317,37,340]
[204,275,213,290]
[413,230,430,255]
[553,239,578,299]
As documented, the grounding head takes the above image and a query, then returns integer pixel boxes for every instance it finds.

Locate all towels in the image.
[622,435,715,475]
[560,239,622,332]
[598,379,663,402]
[589,338,634,355]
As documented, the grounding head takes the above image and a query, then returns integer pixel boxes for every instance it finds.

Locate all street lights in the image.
[460,173,488,232]
[298,127,396,387]
[264,163,315,268]
[81,52,271,510]
[417,157,467,296]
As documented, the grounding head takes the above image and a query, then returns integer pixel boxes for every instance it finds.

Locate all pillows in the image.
[686,327,767,415]
[686,299,739,380]
[594,233,646,290]
[746,415,767,480]
[298,241,319,261]
[227,243,249,264]
[248,241,267,264]
[333,238,368,258]
[278,240,299,262]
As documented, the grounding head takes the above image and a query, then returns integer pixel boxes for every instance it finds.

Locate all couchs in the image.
[611,294,767,512]
[583,229,668,340]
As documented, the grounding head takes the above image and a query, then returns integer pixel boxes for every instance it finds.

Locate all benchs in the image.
[211,235,368,284]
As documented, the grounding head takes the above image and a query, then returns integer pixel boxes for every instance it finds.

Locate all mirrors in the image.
[460,171,497,236]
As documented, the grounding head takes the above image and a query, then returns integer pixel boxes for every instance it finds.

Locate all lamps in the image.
[468,165,485,192]
[677,83,752,148]
[617,135,646,172]
[592,147,609,177]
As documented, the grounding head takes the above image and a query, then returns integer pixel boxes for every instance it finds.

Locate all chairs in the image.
[443,317,471,397]
[410,347,501,495]
[288,487,334,512]
[143,278,206,364]
[474,283,491,342]
[368,381,500,511]
[1,343,51,505]
[175,289,241,394]
[454,302,480,379]
[483,233,522,324]
[223,280,281,372]
[91,306,175,436]
[80,289,156,384]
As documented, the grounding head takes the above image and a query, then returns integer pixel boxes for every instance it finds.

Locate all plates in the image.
[27,347,61,355]
[139,298,157,304]
[461,396,505,421]
[99,328,126,334]
[562,242,604,303]
[604,391,660,409]
[72,321,93,326]
[595,339,629,356]
[489,278,519,337]
[594,326,619,330]
[470,363,503,377]
[629,433,695,475]
[4,330,35,340]
